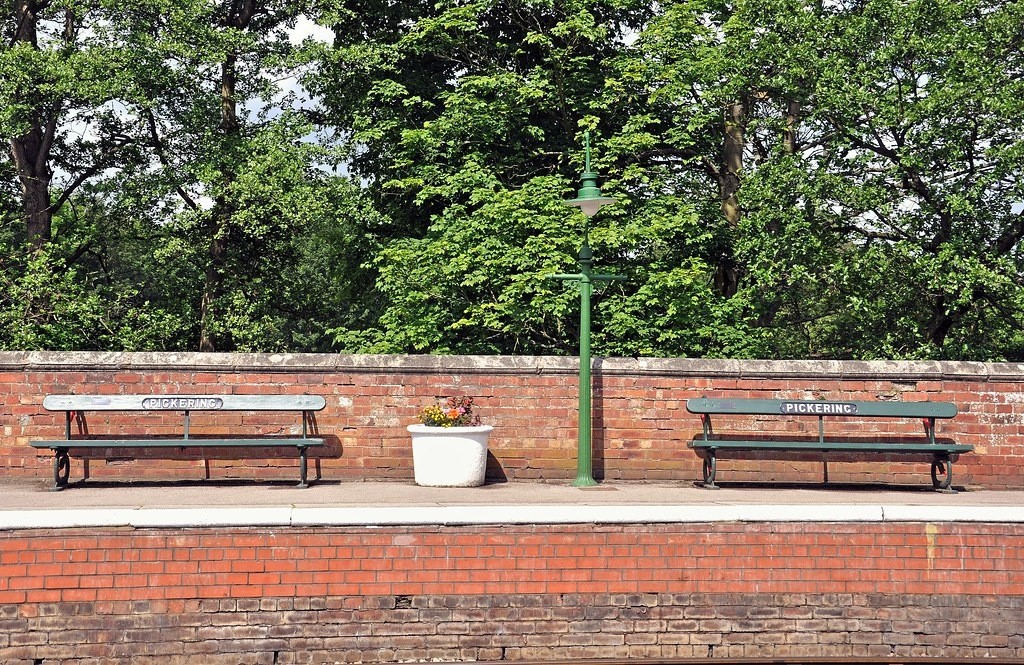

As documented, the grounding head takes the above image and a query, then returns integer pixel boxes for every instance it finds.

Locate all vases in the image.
[407,424,494,486]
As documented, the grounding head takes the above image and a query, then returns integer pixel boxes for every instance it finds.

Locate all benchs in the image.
[31,392,330,491]
[684,400,973,489]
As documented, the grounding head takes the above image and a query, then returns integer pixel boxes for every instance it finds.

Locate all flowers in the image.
[419,395,480,427]
